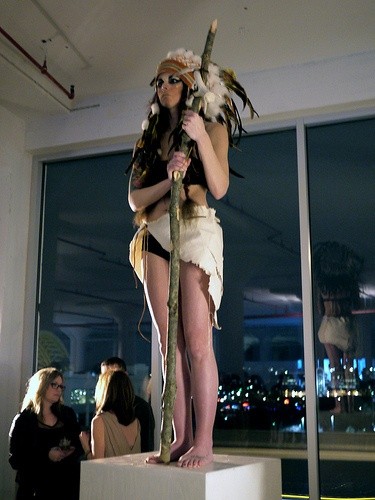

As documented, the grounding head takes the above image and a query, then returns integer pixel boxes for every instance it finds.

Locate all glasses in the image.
[46,380,67,390]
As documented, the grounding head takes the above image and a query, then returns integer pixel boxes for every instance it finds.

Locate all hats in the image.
[155,45,260,141]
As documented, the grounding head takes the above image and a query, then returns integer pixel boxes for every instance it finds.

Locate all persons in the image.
[79,368,141,459]
[318,241,360,415]
[8,367,85,500]
[100,357,155,453]
[127,48,230,468]
